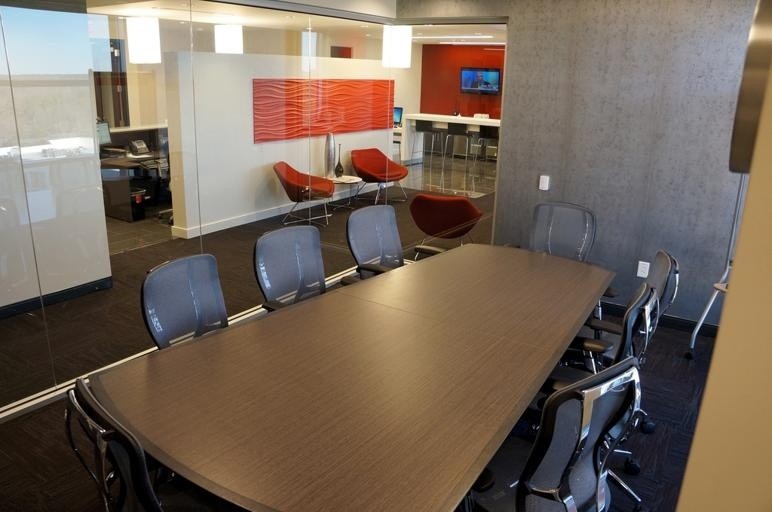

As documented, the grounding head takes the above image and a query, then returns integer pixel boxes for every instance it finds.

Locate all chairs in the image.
[273,148,409,228]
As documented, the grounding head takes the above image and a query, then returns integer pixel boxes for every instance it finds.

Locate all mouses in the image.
[394,126,398,128]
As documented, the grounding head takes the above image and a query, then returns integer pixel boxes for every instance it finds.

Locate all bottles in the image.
[335,143,343,178]
[323,131,334,178]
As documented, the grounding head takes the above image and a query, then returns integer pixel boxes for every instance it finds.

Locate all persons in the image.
[470,72,493,88]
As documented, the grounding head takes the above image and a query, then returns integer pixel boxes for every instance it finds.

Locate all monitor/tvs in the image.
[393,107,403,124]
[97,121,112,146]
[461,67,500,95]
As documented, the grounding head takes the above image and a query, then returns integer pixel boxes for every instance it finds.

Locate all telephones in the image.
[129,139,150,155]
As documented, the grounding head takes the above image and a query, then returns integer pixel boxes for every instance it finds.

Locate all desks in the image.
[100,146,168,224]
[402,112,502,197]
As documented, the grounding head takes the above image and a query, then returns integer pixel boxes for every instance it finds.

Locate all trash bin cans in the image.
[129,187,147,221]
